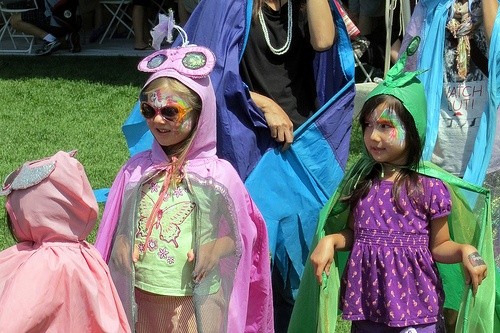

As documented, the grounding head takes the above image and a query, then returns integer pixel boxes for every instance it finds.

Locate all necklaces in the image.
[257,0,293,55]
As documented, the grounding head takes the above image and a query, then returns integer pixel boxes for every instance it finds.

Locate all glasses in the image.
[140,102,192,120]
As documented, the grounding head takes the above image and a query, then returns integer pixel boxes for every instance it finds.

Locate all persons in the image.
[95,8,274,333]
[0,149,131,333]
[0,0,201,55]
[288,36,500,333]
[391,0,500,209]
[123,0,356,333]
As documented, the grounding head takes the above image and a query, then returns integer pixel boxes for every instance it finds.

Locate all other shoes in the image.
[35,40,63,56]
[65,33,82,53]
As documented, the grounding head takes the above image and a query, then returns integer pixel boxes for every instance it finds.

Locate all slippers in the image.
[134,44,153,51]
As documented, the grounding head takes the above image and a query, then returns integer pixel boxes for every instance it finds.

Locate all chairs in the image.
[96,0,135,44]
[0,0,41,52]
